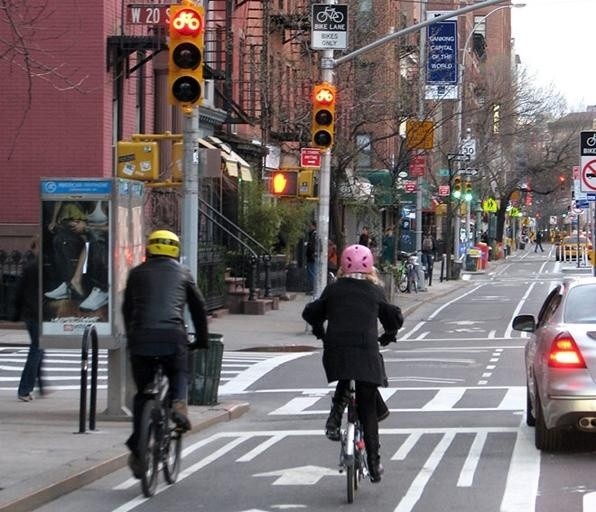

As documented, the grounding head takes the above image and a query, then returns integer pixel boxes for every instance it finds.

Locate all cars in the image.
[556,234,593,263]
[511,274,596,453]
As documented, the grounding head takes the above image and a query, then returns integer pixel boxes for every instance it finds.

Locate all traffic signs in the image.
[455,167,479,177]
[459,136,476,161]
[445,152,471,163]
[127,4,169,25]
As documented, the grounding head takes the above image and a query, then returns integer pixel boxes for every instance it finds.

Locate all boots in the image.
[363,431,385,484]
[325,388,351,443]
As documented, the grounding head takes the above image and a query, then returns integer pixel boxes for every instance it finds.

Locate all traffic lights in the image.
[463,181,473,203]
[267,163,315,202]
[561,177,566,191]
[451,174,462,200]
[308,78,338,153]
[165,0,204,118]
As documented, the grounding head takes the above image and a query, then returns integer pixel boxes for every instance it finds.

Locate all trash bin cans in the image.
[451,262,462,280]
[489,247,493,262]
[520,243,525,249]
[187,333,224,405]
[504,247,507,257]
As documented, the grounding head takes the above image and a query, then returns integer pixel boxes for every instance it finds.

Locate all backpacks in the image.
[422,234,433,250]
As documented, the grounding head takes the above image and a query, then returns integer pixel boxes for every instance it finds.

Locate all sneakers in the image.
[44,281,74,300]
[128,452,151,480]
[168,399,192,432]
[78,287,109,313]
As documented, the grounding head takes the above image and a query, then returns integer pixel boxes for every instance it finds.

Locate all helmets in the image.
[338,243,375,275]
[146,228,181,259]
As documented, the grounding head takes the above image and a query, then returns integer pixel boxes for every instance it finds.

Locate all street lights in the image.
[452,2,528,265]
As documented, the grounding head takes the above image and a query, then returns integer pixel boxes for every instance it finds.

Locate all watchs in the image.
[83,220,90,233]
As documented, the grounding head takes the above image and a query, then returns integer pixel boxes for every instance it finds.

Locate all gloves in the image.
[188,333,209,351]
[378,332,397,346]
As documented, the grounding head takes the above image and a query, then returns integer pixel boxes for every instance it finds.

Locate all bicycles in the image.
[397,248,422,294]
[134,339,212,499]
[310,333,397,507]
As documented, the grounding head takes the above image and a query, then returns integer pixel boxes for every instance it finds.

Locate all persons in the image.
[11,234,52,402]
[423,232,437,286]
[383,227,393,263]
[535,231,544,253]
[302,244,400,484]
[120,229,209,479]
[50,200,109,311]
[303,220,317,296]
[358,226,370,246]
